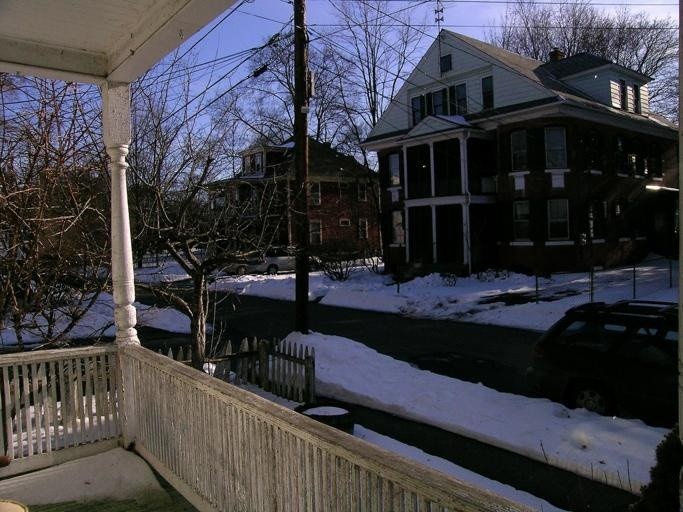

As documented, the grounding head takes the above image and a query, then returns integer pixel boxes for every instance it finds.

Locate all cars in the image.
[222,245,323,275]
